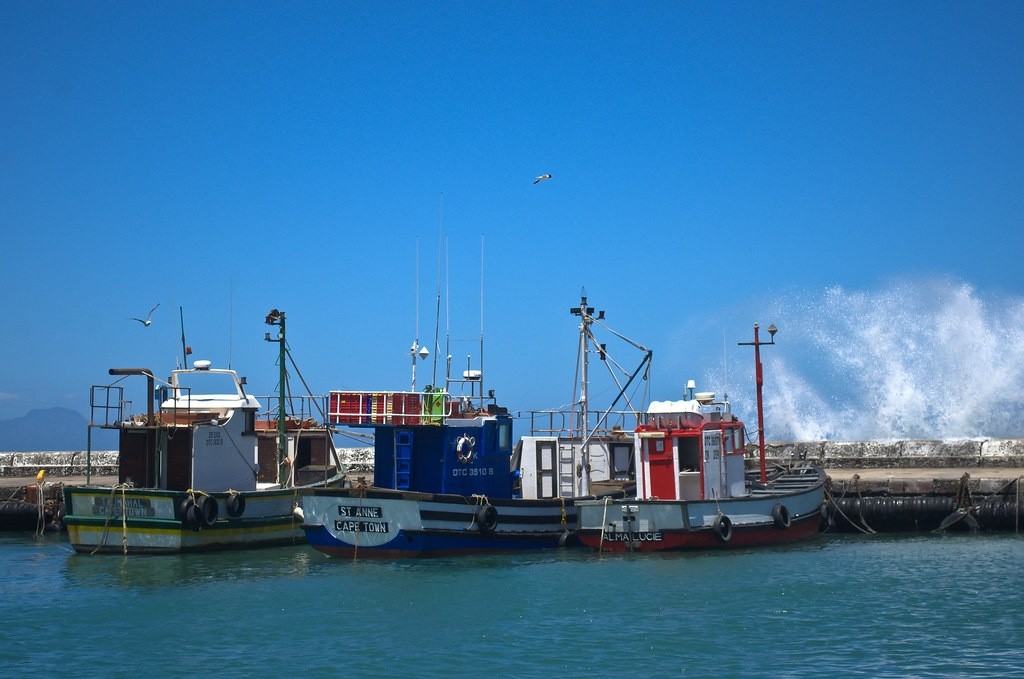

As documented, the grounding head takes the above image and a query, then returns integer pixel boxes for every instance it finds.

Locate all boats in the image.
[290,196,636,558]
[564,284,829,554]
[54,300,374,554]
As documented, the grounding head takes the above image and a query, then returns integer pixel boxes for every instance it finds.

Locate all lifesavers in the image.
[558,531,577,547]
[196,495,219,527]
[773,504,792,530]
[478,505,499,534]
[456,437,474,465]
[940,510,980,532]
[820,503,830,522]
[180,499,201,532]
[226,492,246,518]
[712,515,735,543]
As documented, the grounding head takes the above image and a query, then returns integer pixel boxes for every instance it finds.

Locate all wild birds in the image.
[130,303,160,327]
[532,173,554,184]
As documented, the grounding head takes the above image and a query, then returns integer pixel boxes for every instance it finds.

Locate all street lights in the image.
[738,321,779,486]
